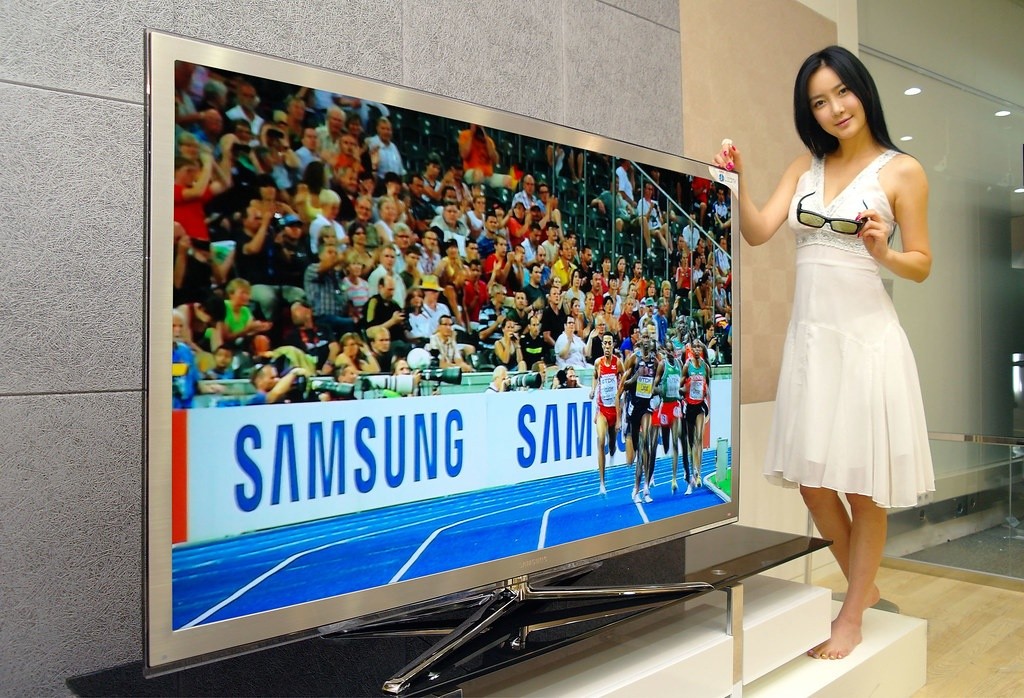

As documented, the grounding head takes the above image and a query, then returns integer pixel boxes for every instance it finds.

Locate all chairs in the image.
[387,107,730,363]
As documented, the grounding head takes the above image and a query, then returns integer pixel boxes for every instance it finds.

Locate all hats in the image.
[645,298,657,307]
[417,275,445,292]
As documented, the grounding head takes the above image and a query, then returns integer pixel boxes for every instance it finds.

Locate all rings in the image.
[720,136,735,146]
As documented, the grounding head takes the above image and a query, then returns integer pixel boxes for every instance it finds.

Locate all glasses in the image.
[797,192,870,236]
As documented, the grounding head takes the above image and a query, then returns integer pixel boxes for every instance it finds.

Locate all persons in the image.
[171,68,510,156]
[587,329,718,507]
[174,283,578,402]
[174,159,553,282]
[557,153,732,326]
[711,44,936,660]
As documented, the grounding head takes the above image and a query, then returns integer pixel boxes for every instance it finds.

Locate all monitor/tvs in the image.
[141,28,742,696]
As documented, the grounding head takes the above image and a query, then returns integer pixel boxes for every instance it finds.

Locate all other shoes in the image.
[696,477,703,487]
[684,485,693,496]
[672,479,680,491]
[632,489,643,503]
[599,485,607,495]
[650,477,655,487]
[644,486,654,503]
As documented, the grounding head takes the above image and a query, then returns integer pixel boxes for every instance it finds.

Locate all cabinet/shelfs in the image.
[66,523,930,698]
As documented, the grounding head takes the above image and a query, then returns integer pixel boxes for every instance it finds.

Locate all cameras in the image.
[354,374,414,395]
[295,374,355,401]
[418,366,461,384]
[506,373,542,392]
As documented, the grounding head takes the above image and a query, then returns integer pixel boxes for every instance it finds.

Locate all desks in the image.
[187,364,732,407]
